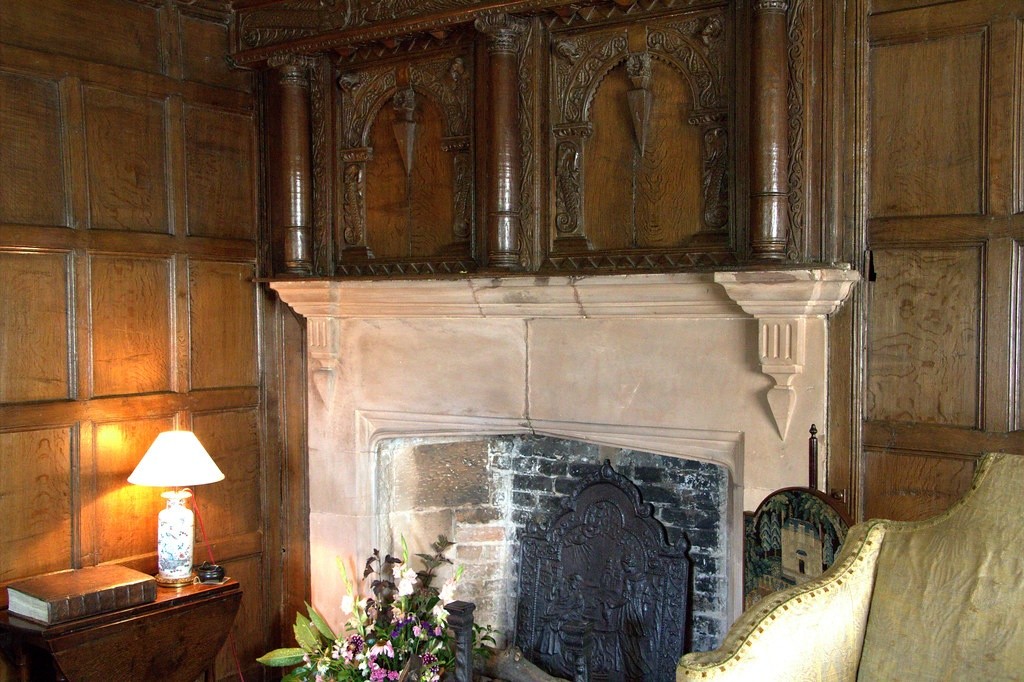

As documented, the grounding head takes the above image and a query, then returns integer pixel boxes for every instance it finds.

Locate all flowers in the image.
[256,534,502,682]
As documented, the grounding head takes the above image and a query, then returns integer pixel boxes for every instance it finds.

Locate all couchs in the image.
[675,453,1024,682]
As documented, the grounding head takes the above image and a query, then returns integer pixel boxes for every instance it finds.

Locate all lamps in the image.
[126,430,225,588]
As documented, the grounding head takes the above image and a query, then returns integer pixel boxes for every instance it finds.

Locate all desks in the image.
[0,570,244,682]
